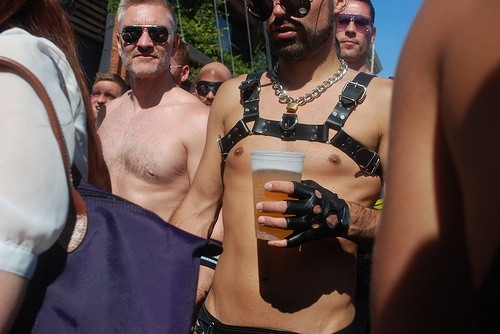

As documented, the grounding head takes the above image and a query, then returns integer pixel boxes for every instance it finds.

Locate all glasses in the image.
[119,23,174,45]
[337,13,373,35]
[247,0,313,23]
[194,80,224,96]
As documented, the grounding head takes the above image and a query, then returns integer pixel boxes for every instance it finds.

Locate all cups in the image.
[250,150,306,242]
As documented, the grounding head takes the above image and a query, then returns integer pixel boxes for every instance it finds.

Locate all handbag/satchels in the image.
[0,55,210,334]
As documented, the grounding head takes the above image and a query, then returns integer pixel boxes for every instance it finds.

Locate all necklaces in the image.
[271,56,348,129]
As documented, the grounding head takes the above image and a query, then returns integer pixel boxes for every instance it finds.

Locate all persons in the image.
[0,0,90,334]
[335,0,376,76]
[83,1,225,304]
[89,41,231,122]
[167,0,394,334]
[370,0,500,334]
